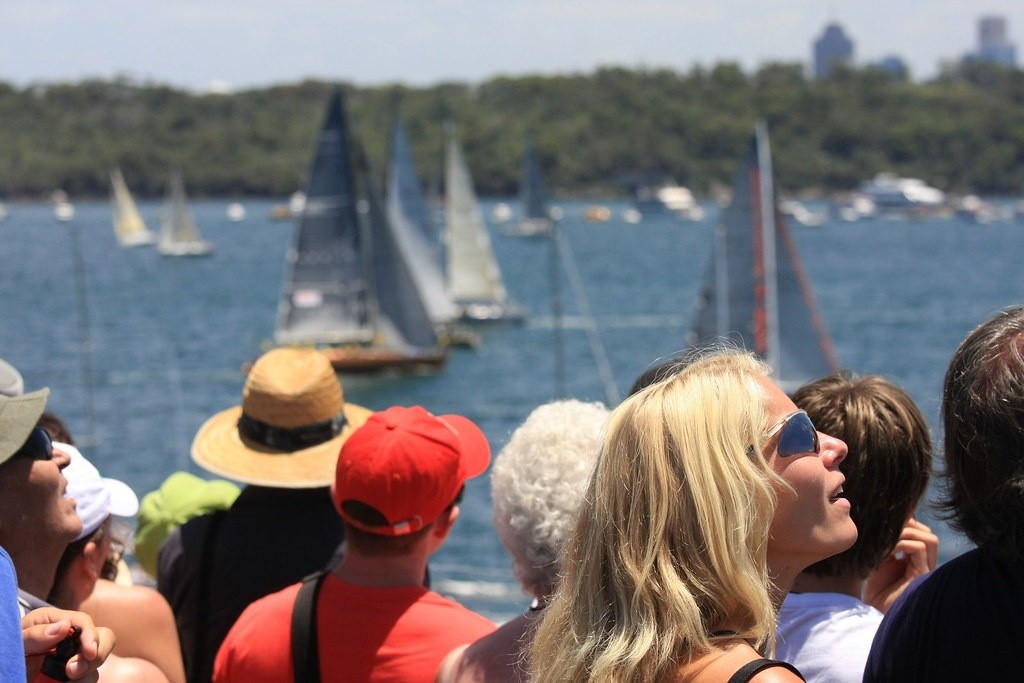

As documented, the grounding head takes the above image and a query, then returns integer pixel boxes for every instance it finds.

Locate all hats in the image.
[331,406,489,535]
[0,357,24,397]
[1,387,51,468]
[190,346,378,488]
[49,439,139,542]
[134,471,241,578]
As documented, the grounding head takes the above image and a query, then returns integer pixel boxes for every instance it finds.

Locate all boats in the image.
[495,168,984,242]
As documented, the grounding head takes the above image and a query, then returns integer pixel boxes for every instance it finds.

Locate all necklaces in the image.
[682,629,762,656]
[528,605,548,611]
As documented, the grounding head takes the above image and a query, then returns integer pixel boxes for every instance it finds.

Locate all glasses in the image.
[745,408,822,458]
[8,428,52,462]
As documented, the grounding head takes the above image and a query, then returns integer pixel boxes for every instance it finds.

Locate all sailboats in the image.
[441,123,530,327]
[237,81,450,379]
[380,104,482,352]
[101,158,157,248]
[156,169,211,260]
[681,123,845,400]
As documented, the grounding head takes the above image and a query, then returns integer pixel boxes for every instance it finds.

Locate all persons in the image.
[0,386,117,683]
[214,403,499,682]
[40,416,185,683]
[762,375,938,683]
[49,441,169,683]
[156,346,376,683]
[434,398,612,683]
[522,354,858,683]
[861,305,1024,683]
